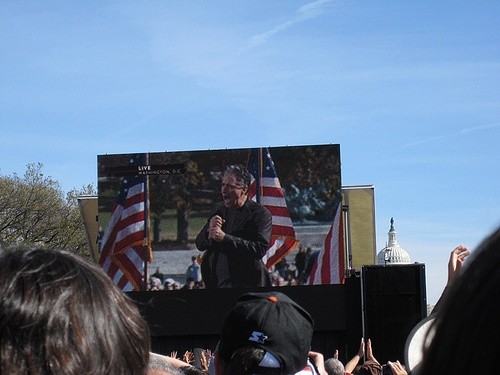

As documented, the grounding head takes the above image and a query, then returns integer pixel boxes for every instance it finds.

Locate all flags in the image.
[301,189,345,285]
[100,153,155,292]
[246,147,300,270]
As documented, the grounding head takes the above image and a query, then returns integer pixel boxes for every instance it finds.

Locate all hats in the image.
[217,292,315,375]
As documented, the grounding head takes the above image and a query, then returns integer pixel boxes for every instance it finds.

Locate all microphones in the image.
[208,209,223,246]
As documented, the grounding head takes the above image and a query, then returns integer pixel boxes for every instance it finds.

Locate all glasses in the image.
[219,181,245,190]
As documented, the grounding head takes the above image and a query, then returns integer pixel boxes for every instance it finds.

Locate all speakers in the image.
[360,264,427,365]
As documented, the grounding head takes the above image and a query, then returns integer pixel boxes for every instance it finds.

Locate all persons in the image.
[411,225,500,375]
[195,164,273,288]
[214,291,314,375]
[165,336,413,375]
[133,246,320,291]
[0,246,150,375]
